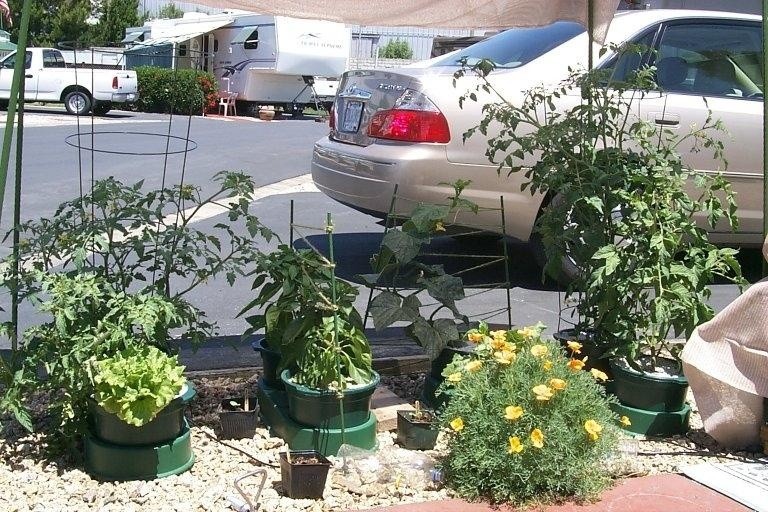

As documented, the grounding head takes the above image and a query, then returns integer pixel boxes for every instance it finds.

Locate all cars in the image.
[312,8,766,285]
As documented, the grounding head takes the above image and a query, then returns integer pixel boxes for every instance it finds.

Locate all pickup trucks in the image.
[0,48,139,116]
